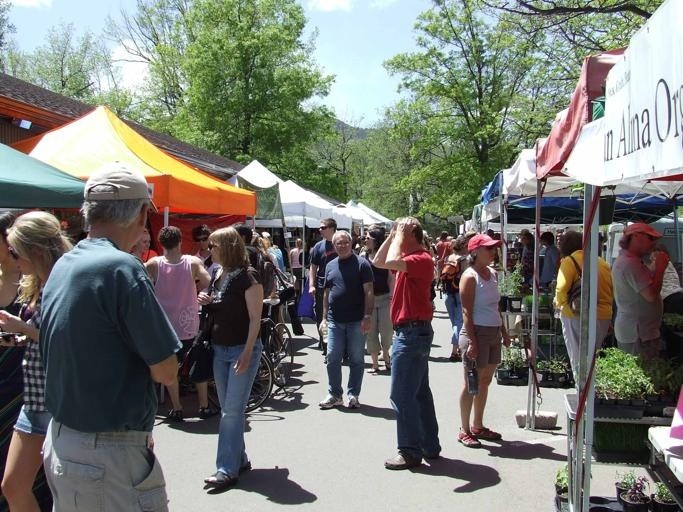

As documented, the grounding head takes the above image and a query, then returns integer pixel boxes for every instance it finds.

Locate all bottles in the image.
[467,368,478,394]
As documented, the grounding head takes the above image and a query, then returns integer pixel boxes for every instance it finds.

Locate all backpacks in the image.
[567,256,581,313]
[440,256,466,293]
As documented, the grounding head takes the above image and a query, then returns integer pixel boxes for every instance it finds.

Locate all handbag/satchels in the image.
[275,285,295,306]
[189,341,213,383]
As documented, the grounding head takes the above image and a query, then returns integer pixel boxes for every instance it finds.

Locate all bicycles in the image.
[208,297,292,413]
[439,264,447,299]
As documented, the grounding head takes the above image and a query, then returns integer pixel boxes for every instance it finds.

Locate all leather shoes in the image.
[384,455,421,469]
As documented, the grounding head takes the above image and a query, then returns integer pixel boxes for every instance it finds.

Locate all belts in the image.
[396,320,430,329]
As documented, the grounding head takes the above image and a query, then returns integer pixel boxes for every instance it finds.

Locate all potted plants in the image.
[555,464,592,512]
[508,263,523,312]
[620,476,652,512]
[497,275,508,312]
[615,469,639,503]
[651,480,679,512]
[592,347,683,454]
[495,344,574,386]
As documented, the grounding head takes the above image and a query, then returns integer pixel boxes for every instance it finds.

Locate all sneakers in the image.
[458,427,501,447]
[200,405,217,419]
[449,349,460,362]
[319,395,343,408]
[348,395,360,408]
[385,356,391,369]
[168,408,182,421]
[367,363,377,373]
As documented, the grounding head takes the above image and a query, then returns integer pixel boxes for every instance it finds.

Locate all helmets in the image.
[468,234,502,251]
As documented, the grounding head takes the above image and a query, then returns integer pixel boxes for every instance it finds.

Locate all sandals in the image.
[205,460,251,485]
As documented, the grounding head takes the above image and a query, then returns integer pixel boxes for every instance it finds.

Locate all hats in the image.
[625,223,663,238]
[84,161,158,213]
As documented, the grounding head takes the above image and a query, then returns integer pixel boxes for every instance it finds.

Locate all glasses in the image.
[208,243,218,250]
[367,236,375,240]
[320,226,328,229]
[195,237,208,241]
[8,247,20,259]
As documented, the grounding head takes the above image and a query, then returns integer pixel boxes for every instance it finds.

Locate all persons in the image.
[285,218,391,374]
[318,229,374,408]
[650,242,683,316]
[613,221,670,356]
[456,234,512,448]
[0,208,53,512]
[552,230,614,393]
[0,210,74,512]
[372,215,442,471]
[133,222,286,425]
[425,228,559,362]
[194,227,265,486]
[40,168,183,512]
